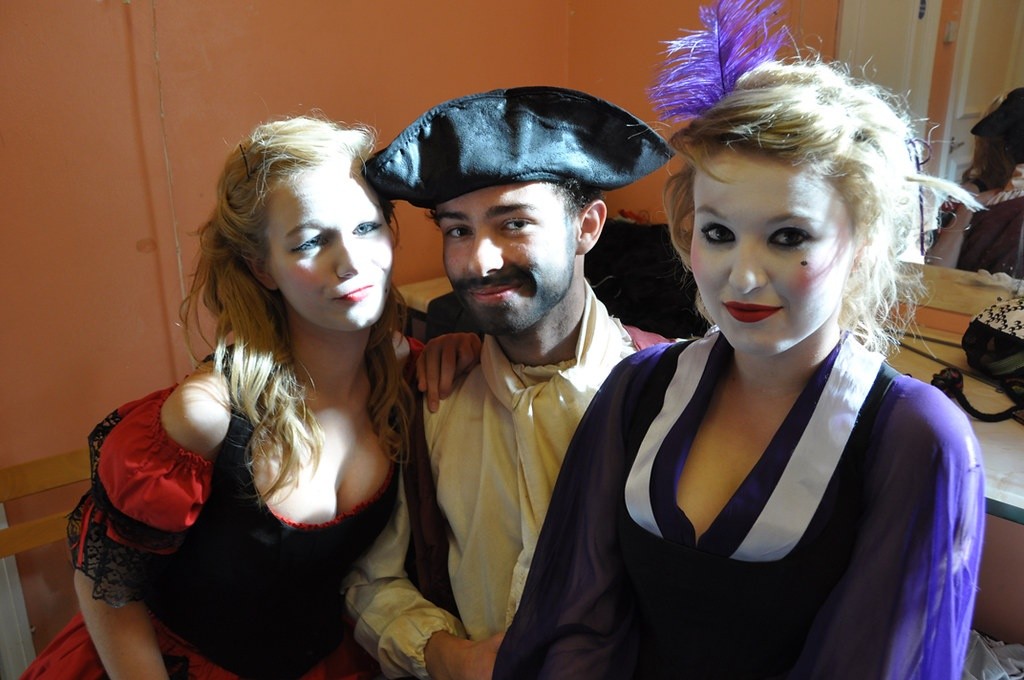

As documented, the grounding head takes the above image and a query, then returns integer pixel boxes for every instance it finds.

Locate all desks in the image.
[883,322,1023,524]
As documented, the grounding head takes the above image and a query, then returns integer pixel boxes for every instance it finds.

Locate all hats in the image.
[970,87,1024,137]
[362,85,678,211]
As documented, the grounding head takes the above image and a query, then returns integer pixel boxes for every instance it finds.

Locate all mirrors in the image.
[833,0,1024,315]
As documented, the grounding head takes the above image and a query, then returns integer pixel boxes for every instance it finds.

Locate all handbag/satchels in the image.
[961,295,1024,385]
[585,219,712,339]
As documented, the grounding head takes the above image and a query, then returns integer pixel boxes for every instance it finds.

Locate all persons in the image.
[925,87,1023,277]
[17,0,990,679]
